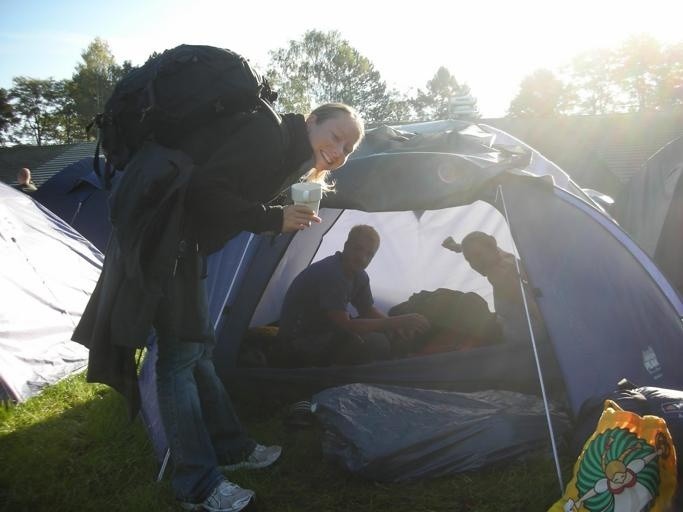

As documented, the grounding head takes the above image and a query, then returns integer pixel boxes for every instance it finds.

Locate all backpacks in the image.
[94,43,288,166]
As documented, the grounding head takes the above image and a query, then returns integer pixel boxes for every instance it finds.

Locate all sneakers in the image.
[217,442,282,471]
[180,478,254,511]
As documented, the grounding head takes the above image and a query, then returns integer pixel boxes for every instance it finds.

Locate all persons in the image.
[441,230,544,341]
[280,221,429,366]
[72,101,364,511]
[11,166,38,195]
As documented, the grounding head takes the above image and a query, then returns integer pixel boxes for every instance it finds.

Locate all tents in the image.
[605,130,683,298]
[199,119,682,478]
[28,154,124,253]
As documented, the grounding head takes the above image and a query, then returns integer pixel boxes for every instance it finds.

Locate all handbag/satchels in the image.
[547,396,677,511]
[570,376,683,460]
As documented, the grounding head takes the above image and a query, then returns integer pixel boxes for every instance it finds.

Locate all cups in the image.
[290,182,323,216]
